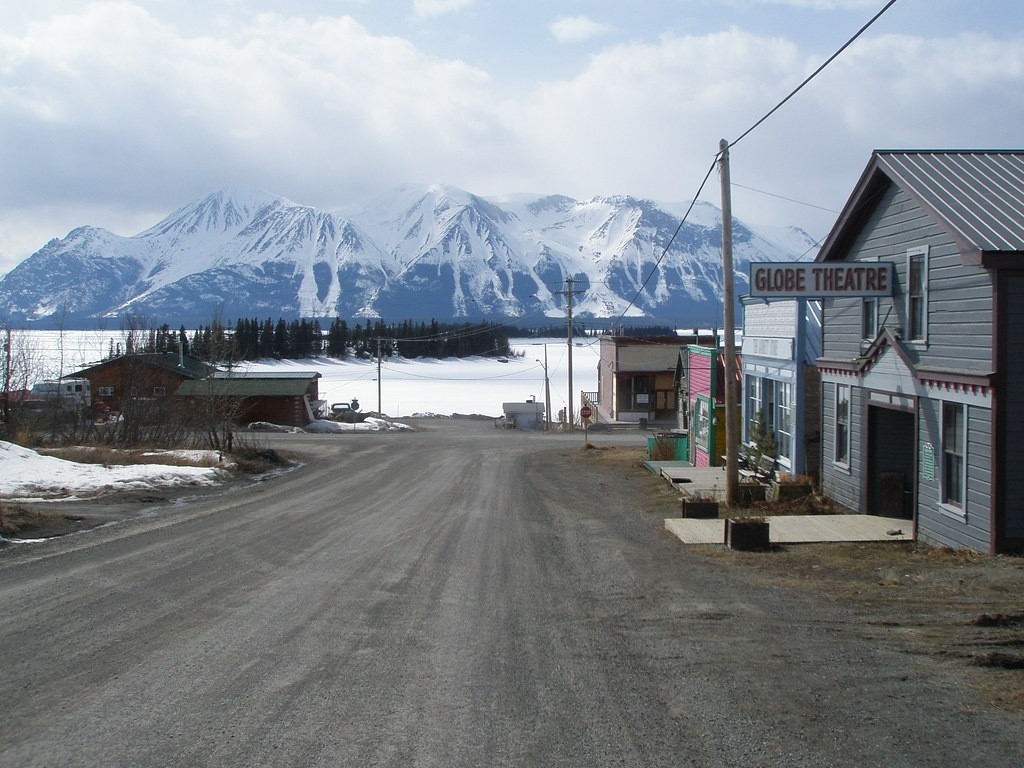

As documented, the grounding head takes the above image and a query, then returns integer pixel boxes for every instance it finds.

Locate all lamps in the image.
[850,339,885,362]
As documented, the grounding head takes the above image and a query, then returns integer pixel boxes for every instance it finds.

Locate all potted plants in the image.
[738,478,766,502]
[723,502,769,550]
[773,471,812,500]
[681,491,719,519]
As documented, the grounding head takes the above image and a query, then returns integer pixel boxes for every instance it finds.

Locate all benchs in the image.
[720,443,749,471]
[738,454,776,488]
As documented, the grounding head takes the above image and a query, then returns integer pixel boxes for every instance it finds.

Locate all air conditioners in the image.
[154,387,168,396]
[98,387,114,396]
